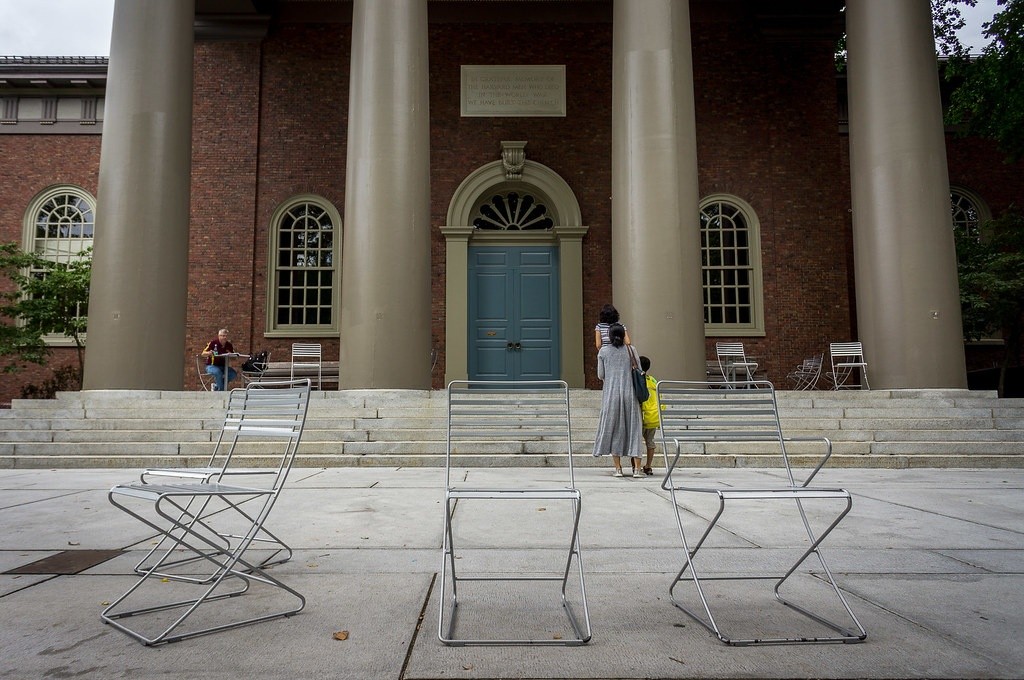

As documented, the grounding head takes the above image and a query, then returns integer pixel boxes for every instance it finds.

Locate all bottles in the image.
[213,344,218,356]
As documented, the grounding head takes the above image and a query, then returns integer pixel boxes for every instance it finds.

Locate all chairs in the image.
[653,378,869,644]
[242,354,272,388]
[290,342,323,390]
[135,387,304,583]
[715,342,759,389]
[438,377,594,645]
[194,354,215,391]
[788,353,823,390]
[103,373,313,645]
[822,353,856,389]
[830,342,872,390]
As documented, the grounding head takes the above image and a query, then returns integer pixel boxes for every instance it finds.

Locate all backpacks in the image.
[241,350,268,372]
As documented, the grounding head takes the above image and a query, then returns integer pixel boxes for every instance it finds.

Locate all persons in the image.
[595,304,631,351]
[201,329,238,391]
[631,355,663,476]
[592,323,649,478]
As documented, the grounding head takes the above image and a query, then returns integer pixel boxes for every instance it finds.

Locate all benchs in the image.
[244,360,338,390]
[705,360,768,389]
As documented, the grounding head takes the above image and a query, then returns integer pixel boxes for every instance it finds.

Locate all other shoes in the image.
[612,466,653,478]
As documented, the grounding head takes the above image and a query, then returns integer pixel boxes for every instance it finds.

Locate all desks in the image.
[214,352,251,392]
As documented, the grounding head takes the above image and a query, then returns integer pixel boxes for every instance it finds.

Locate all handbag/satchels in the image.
[632,366,650,403]
